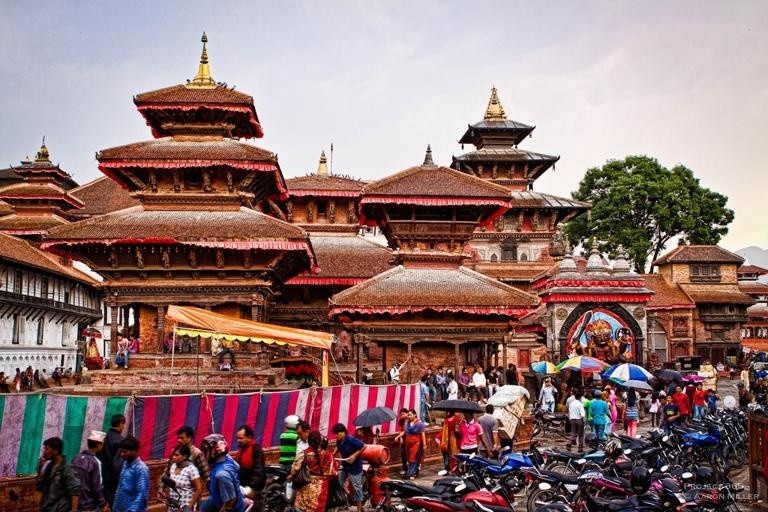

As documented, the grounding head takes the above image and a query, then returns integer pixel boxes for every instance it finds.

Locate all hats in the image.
[87,430,107,444]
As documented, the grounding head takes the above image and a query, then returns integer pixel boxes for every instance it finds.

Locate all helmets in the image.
[283,415,300,429]
[198,434,229,462]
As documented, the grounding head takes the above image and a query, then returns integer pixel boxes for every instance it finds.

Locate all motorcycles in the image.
[259,461,288,512]
[154,477,258,512]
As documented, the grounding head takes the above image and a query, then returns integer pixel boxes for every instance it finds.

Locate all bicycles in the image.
[326,453,376,512]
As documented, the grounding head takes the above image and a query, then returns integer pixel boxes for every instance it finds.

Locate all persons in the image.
[0,329,767,512]
